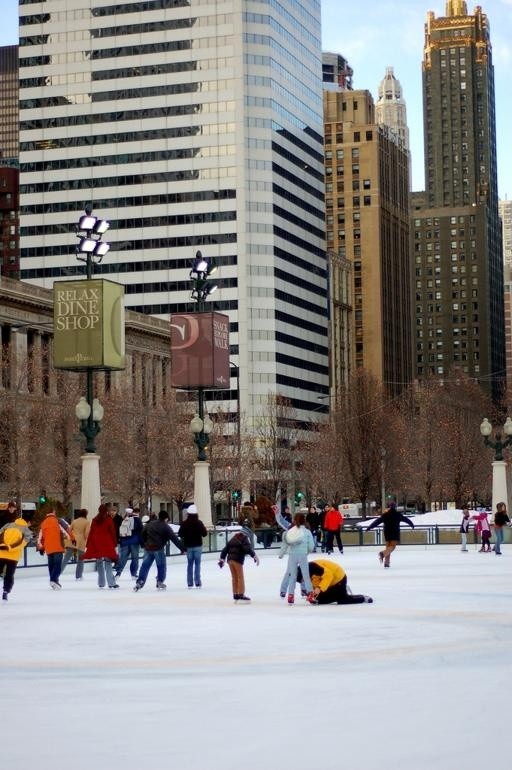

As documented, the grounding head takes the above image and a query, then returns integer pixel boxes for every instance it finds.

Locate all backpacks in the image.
[119,516,138,536]
[285,525,304,545]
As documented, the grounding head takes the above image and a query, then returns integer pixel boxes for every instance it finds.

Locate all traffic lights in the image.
[40,491,48,509]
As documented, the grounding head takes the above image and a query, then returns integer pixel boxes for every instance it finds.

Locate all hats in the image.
[187,504,199,515]
[126,508,134,514]
[479,508,486,514]
[6,500,14,505]
[142,516,150,521]
[389,502,398,508]
[462,510,468,514]
[241,524,254,538]
[159,510,170,519]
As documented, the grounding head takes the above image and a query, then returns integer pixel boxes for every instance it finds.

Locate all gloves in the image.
[218,559,224,568]
[365,528,369,532]
[72,540,77,546]
[272,505,279,513]
[412,527,415,530]
[140,543,144,549]
[182,548,187,553]
[1,546,7,550]
[254,555,259,565]
[39,549,45,556]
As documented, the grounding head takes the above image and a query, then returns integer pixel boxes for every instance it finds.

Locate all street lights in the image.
[169,249,234,537]
[288,430,298,526]
[377,439,387,515]
[480,415,512,529]
[50,197,129,520]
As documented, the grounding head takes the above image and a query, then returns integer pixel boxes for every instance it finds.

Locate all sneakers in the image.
[479,546,502,554]
[302,590,306,596]
[237,593,250,601]
[99,572,166,589]
[383,564,390,568]
[188,584,202,588]
[288,594,295,603]
[234,593,238,599]
[378,552,384,563]
[306,591,318,604]
[313,550,334,555]
[364,595,372,603]
[50,580,62,589]
[280,592,287,597]
[3,592,8,600]
[341,550,343,553]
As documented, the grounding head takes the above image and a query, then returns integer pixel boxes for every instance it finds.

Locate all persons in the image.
[1,501,31,605]
[459,509,474,552]
[472,506,493,553]
[217,505,374,606]
[365,501,416,567]
[491,502,512,555]
[36,502,207,589]
